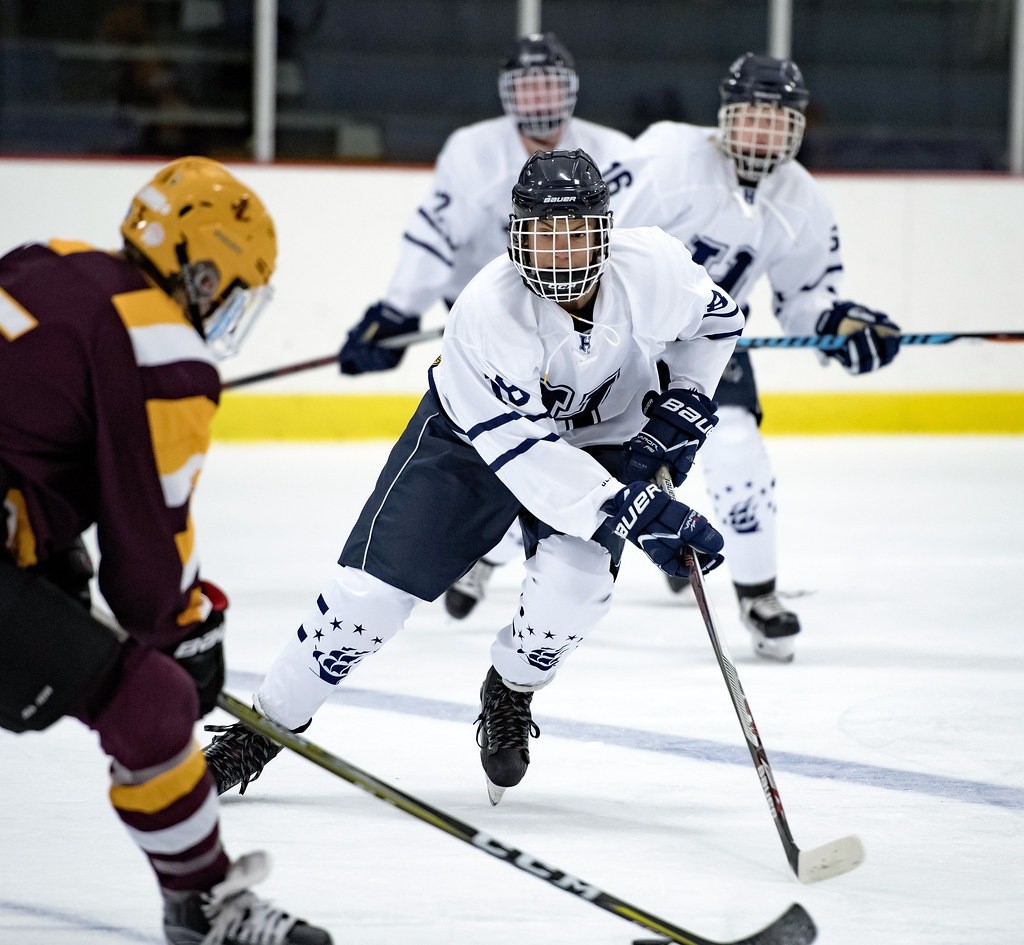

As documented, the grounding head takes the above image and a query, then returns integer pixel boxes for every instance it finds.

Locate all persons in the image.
[0,158,333,945]
[199,150,745,806]
[444,53,901,664]
[339,33,693,593]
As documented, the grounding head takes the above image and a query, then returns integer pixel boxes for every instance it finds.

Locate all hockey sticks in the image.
[218,322,443,395]
[734,331,1024,345]
[657,461,867,883]
[214,691,820,945]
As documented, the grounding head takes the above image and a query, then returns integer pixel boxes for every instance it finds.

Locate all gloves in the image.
[618,388,719,488]
[141,580,231,719]
[603,479,724,580]
[815,299,900,376]
[334,302,418,375]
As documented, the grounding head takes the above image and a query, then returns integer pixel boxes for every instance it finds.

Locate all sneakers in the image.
[471,665,540,806]
[736,593,801,662]
[441,558,506,629]
[200,706,312,797]
[162,850,332,945]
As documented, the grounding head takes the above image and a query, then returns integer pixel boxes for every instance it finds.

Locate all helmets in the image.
[717,52,807,182]
[498,32,579,139]
[120,156,278,357]
[506,147,613,304]
